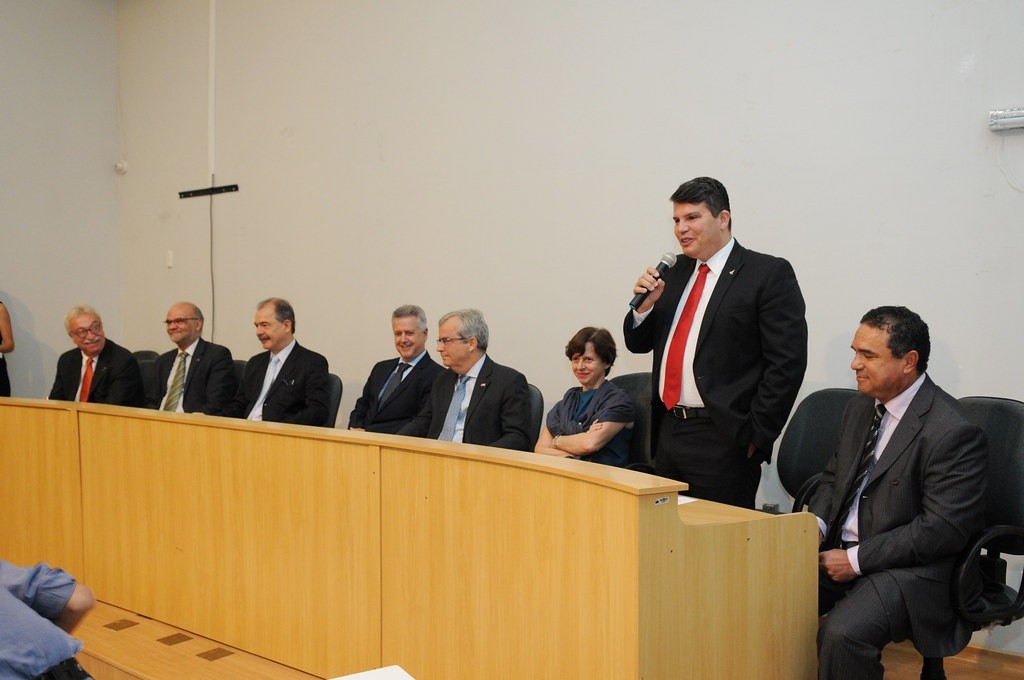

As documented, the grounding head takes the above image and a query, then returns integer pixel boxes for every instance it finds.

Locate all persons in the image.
[141,302,235,416]
[535,327,634,468]
[0,301,14,397]
[48,305,146,407]
[218,297,329,426]
[396,308,532,452]
[0,561,96,680]
[348,304,449,438]
[800,306,988,680]
[624,177,808,510]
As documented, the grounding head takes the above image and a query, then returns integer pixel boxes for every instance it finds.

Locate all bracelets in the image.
[553,435,561,450]
[586,431,589,433]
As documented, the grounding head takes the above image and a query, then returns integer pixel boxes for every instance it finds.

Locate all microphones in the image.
[629,253,677,311]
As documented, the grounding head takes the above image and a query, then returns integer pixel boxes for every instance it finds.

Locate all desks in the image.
[0,397,819,680]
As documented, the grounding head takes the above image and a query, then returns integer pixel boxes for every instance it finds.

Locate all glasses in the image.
[71,322,101,338]
[163,318,199,326]
[436,337,467,346]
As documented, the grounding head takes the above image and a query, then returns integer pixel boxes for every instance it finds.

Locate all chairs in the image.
[795,397,1024,680]
[315,371,343,428]
[609,372,659,472]
[518,381,543,453]
[777,389,864,516]
[223,360,252,416]
[132,351,164,410]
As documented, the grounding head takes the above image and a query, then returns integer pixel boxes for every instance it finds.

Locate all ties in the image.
[377,361,411,411]
[437,375,471,441]
[79,359,94,403]
[822,404,888,553]
[163,352,189,412]
[246,355,280,421]
[661,264,711,412]
